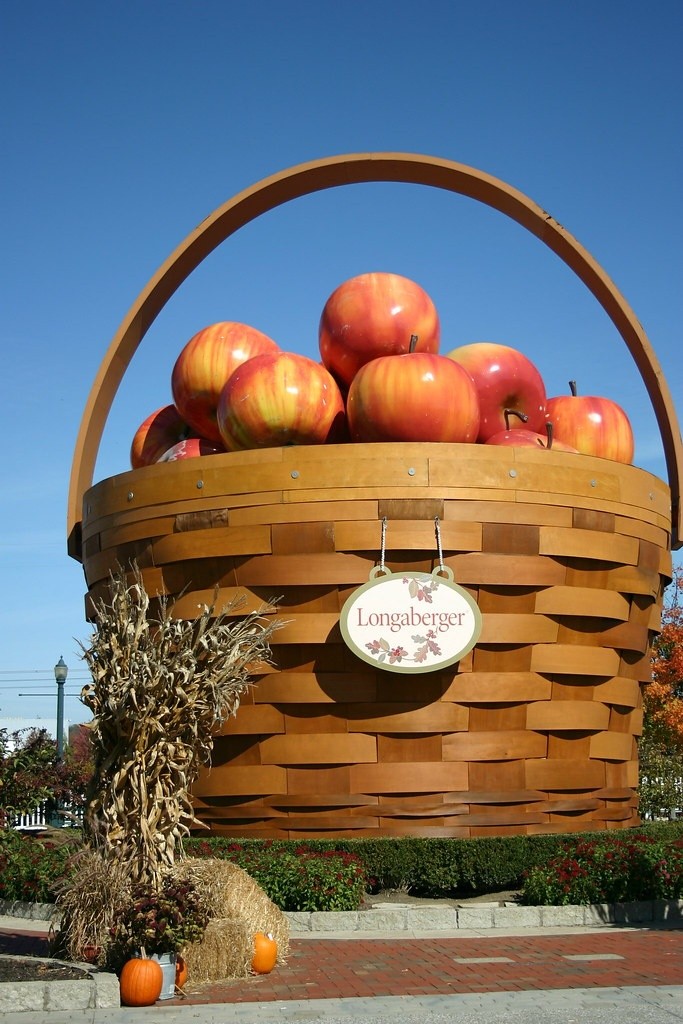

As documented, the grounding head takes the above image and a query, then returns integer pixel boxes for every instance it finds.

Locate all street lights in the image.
[47,654,69,828]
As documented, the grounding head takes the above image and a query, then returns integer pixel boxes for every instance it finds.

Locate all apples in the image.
[129,272,634,466]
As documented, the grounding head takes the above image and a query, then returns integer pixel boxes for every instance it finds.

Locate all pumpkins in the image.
[175,954,187,991]
[119,945,163,1006]
[250,931,277,974]
